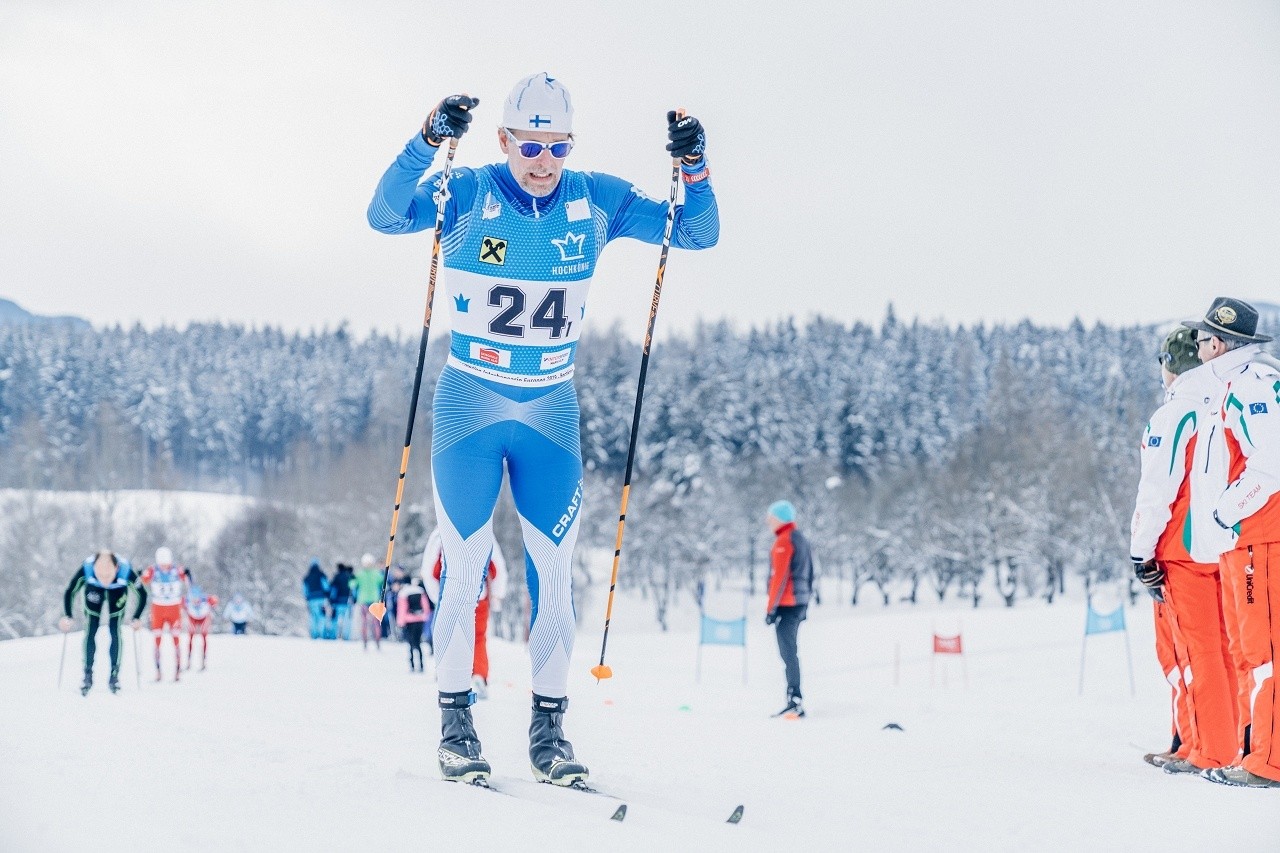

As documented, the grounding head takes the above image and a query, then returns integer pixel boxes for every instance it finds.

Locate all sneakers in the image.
[1210,766,1273,788]
[1200,768,1220,784]
[773,705,805,722]
[1143,754,1176,768]
[1162,761,1202,775]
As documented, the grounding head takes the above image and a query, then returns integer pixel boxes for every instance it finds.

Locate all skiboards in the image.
[437,775,745,827]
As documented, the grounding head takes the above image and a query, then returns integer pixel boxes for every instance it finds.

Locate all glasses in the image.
[505,129,576,160]
[1195,335,1224,351]
[1157,353,1173,366]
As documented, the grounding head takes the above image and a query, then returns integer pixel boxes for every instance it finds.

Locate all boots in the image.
[436,687,492,785]
[527,693,590,787]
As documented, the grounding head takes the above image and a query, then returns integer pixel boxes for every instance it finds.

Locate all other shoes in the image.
[109,669,121,691]
[79,669,93,691]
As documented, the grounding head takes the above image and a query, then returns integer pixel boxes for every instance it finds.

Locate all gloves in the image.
[665,111,708,166]
[422,95,480,148]
[1131,558,1167,605]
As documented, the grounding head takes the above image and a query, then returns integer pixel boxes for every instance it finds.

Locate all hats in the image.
[499,71,575,133]
[156,547,173,565]
[1181,297,1273,342]
[361,553,376,566]
[1162,328,1204,374]
[769,500,796,524]
[401,574,413,584]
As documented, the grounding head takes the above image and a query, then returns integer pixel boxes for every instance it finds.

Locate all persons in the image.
[137,546,219,680]
[59,548,147,693]
[1130,297,1280,789]
[301,522,509,699]
[367,70,721,784]
[765,501,813,718]
[223,594,253,635]
